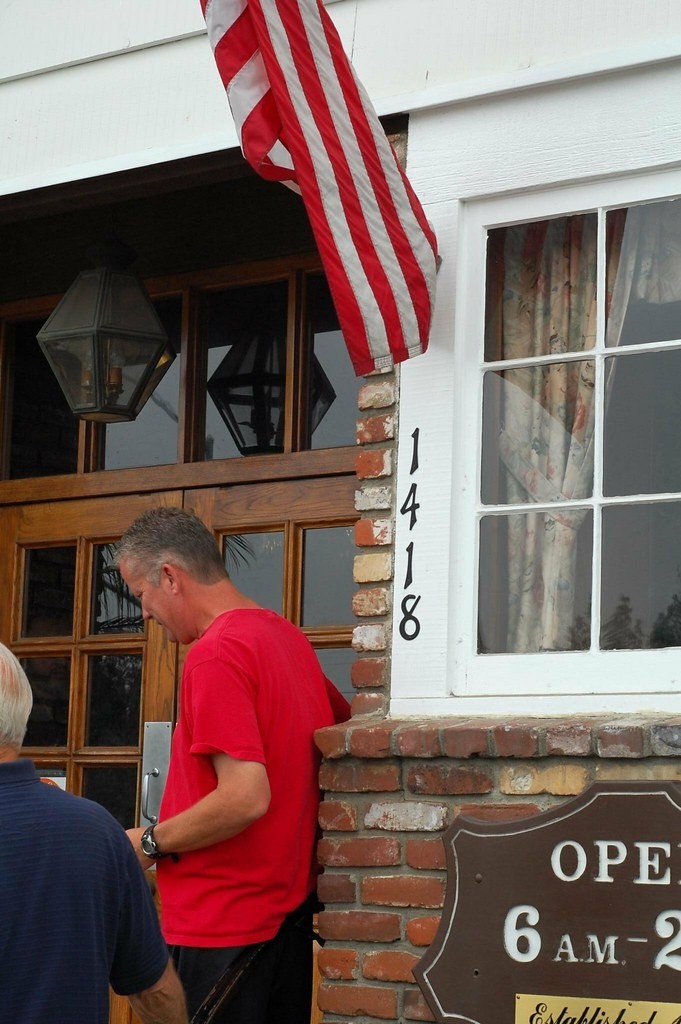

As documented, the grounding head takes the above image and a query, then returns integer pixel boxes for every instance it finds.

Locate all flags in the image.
[200,0,438,375]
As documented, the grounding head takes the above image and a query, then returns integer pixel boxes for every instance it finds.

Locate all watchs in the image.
[141,824,180,863]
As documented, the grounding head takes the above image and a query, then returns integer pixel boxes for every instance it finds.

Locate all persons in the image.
[117,506,352,1024]
[1,641,187,1024]
